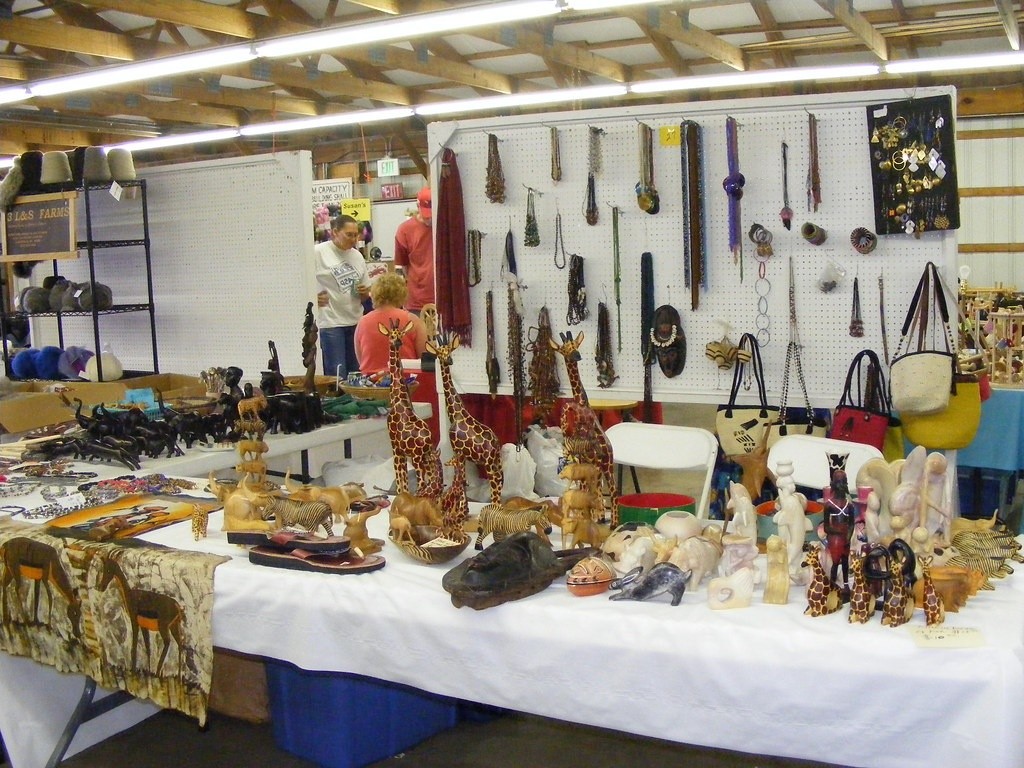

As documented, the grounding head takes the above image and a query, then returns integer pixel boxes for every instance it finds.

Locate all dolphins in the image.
[727,479,817,585]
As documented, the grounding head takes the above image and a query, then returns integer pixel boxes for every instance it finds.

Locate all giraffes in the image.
[801,545,945,628]
[376,316,619,525]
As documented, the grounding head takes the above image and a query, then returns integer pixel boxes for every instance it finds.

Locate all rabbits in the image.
[609,562,693,607]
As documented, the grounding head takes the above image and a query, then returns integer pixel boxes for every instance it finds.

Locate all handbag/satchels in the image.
[714,333,782,456]
[924,261,993,402]
[829,349,890,449]
[886,261,960,417]
[844,350,905,465]
[898,261,982,450]
[764,338,829,455]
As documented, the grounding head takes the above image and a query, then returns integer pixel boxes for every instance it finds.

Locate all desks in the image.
[0,392,433,487]
[0,476,1024,768]
[890,387,1024,519]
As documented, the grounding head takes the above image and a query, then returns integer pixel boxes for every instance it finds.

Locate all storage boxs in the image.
[330,161,366,183]
[956,467,1019,520]
[207,646,269,728]
[0,373,208,433]
[262,653,459,768]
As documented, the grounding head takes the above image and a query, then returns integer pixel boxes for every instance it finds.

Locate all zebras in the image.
[945,531,1024,591]
[475,497,553,551]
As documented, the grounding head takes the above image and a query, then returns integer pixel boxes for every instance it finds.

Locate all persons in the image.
[355,271,429,384]
[393,188,435,320]
[314,215,373,384]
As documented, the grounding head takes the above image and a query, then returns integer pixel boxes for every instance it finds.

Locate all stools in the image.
[585,398,642,496]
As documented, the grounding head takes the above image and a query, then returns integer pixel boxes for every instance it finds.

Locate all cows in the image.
[912,565,986,613]
[612,528,722,589]
[39,389,341,471]
[261,468,385,553]
[205,394,282,534]
[388,494,466,543]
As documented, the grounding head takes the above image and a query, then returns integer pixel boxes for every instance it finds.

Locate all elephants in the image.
[950,509,998,532]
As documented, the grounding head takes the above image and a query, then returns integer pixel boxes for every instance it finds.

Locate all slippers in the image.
[226,528,350,552]
[249,540,388,577]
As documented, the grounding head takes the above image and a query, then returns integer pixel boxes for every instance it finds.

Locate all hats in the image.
[12,274,113,314]
[0,140,140,211]
[11,341,124,382]
[417,185,433,220]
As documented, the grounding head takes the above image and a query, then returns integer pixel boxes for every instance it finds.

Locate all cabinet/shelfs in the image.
[0,178,159,383]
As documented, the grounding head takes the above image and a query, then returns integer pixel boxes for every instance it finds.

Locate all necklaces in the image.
[464,119,864,462]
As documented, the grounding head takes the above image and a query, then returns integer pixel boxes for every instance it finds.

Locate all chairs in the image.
[765,435,885,496]
[602,421,719,521]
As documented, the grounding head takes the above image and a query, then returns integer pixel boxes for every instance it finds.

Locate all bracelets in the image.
[748,223,877,348]
